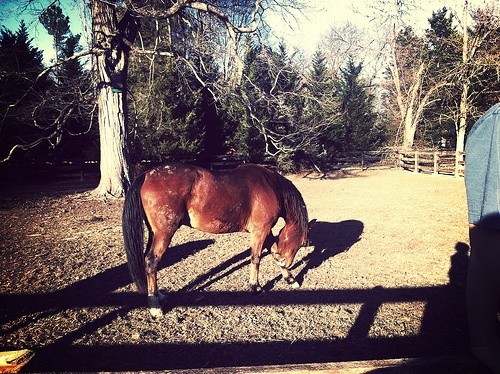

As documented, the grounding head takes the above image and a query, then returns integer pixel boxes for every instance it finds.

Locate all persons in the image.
[465,102,500,374]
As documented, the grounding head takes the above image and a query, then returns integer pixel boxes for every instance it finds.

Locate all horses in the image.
[117,160,318,320]
